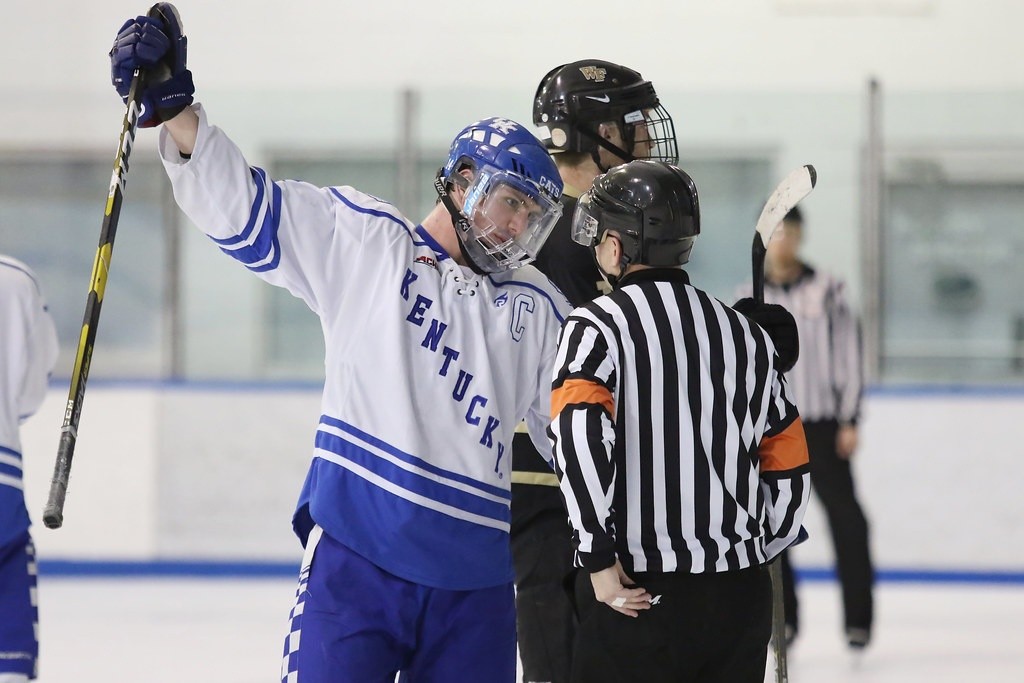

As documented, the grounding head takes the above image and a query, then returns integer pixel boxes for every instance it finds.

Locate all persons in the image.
[0,256,58,683]
[509,57,679,683]
[721,196,876,648]
[108,1,578,683]
[547,160,811,683]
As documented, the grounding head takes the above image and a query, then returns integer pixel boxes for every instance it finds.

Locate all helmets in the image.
[532,59,680,167]
[581,159,702,268]
[444,116,563,211]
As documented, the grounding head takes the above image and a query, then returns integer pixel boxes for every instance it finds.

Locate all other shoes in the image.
[848,626,869,647]
[771,620,794,652]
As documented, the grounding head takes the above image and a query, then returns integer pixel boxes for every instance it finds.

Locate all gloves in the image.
[110,2,195,129]
[733,297,800,375]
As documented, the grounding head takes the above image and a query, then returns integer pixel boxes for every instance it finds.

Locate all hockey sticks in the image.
[747,159,823,683]
[39,0,189,534]
